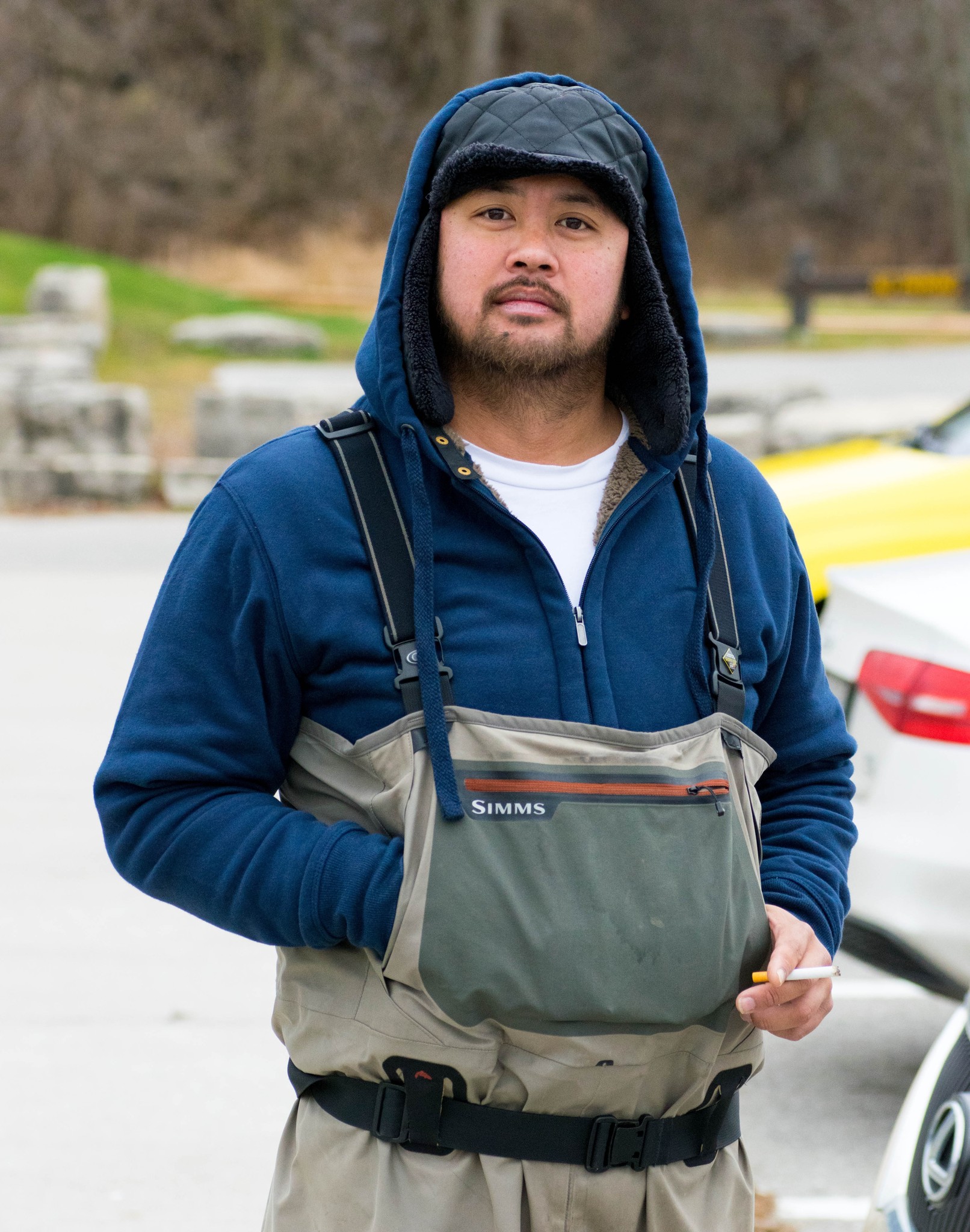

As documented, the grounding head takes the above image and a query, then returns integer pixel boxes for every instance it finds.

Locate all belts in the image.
[285,1057,742,1172]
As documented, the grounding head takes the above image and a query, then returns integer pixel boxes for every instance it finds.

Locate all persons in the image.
[93,72,858,1232]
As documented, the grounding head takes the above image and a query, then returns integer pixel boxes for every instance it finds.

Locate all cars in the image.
[733,394,970,606]
[810,569,970,1005]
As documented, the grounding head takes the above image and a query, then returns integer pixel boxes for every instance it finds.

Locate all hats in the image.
[402,82,690,480]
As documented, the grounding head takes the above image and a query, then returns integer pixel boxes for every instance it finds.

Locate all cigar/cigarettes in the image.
[752,965,841,983]
